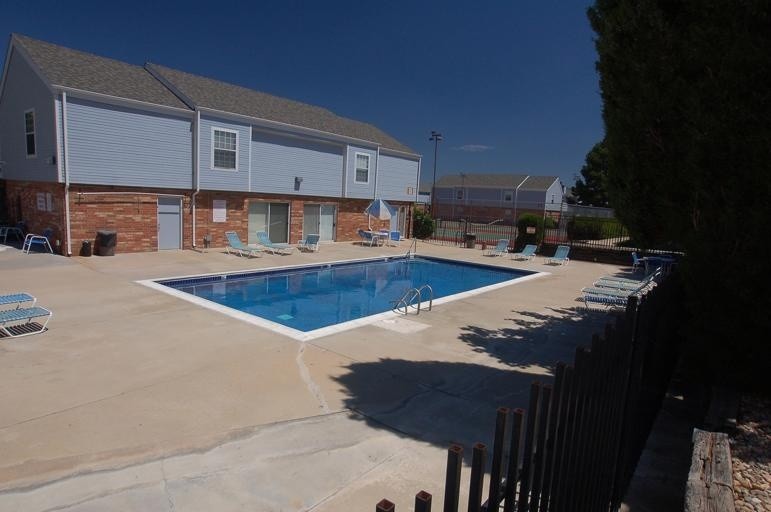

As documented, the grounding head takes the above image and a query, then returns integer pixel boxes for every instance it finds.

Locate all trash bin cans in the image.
[465,233,476,248]
[82,241,91,256]
[98,230,116,256]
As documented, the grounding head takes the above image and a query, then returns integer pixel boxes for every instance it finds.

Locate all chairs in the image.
[224,231,321,260]
[482,239,571,267]
[0,293,53,338]
[0,220,55,255]
[358,229,401,248]
[580,251,673,306]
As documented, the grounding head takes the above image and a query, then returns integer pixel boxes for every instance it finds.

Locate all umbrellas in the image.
[359,278,390,299]
[365,197,397,232]
